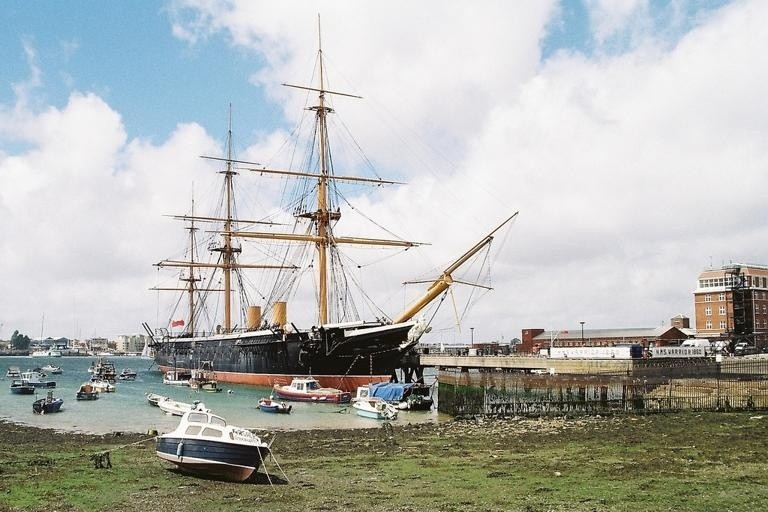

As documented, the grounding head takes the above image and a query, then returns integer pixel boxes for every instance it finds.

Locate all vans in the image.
[680,339,748,356]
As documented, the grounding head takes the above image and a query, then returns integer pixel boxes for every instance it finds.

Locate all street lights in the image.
[470,328,475,349]
[579,321,585,346]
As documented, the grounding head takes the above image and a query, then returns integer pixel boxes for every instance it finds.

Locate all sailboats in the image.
[139,10,522,397]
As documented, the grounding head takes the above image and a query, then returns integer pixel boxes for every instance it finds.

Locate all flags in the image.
[171,320,184,327]
